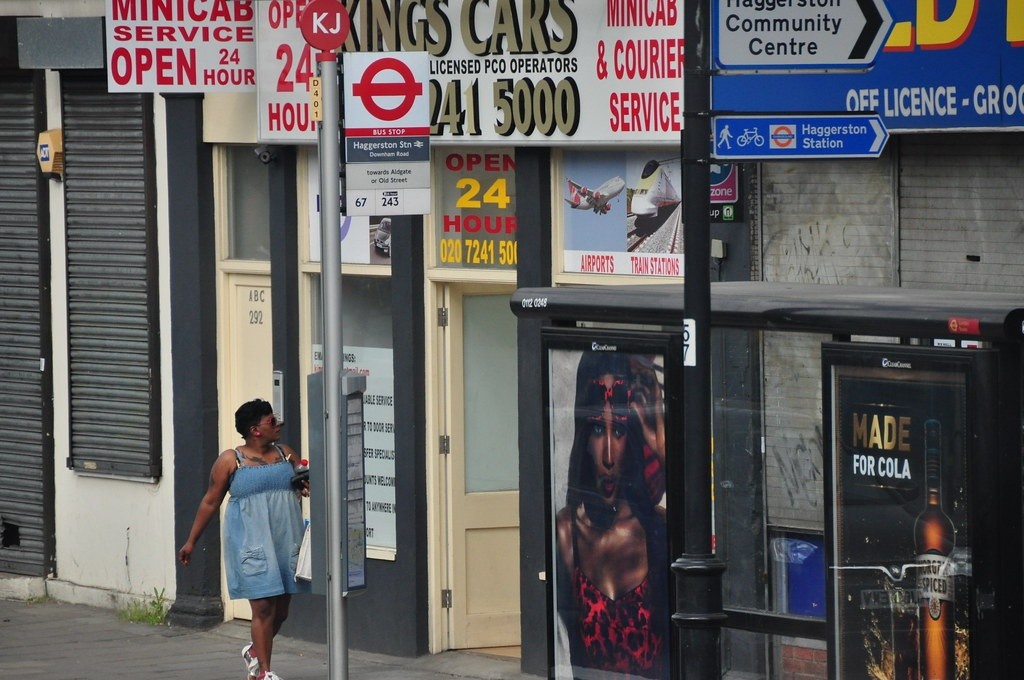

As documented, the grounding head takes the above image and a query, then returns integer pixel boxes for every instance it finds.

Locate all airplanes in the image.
[564,176,625,215]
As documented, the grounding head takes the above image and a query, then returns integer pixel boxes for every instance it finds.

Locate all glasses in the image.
[254,417,276,427]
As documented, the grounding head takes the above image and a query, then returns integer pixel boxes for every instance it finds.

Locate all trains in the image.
[631,160,681,219]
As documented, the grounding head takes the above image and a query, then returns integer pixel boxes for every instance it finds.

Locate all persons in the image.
[555,351,671,680]
[179,399,310,680]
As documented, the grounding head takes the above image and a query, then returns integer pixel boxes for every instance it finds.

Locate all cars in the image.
[373,217,392,254]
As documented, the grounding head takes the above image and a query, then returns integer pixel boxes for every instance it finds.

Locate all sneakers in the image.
[241,642,282,680]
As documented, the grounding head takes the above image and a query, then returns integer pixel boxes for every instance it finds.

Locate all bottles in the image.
[914,417,957,680]
[294,459,309,502]
